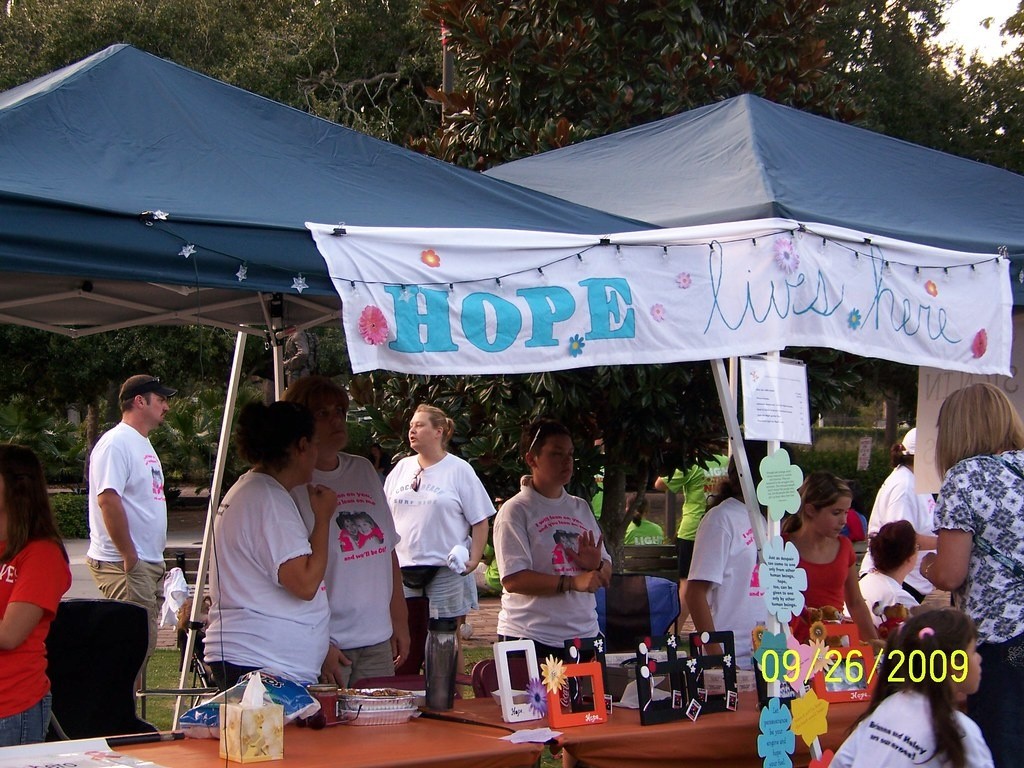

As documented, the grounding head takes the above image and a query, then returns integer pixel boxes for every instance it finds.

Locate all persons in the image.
[624,498,665,571]
[826,609,994,768]
[202,400,339,691]
[921,382,1024,768]
[385,403,497,674]
[842,520,922,631]
[782,470,877,644]
[684,439,780,673]
[492,418,615,663]
[1,442,73,747]
[857,427,937,607]
[655,453,729,577]
[281,374,410,686]
[85,374,170,670]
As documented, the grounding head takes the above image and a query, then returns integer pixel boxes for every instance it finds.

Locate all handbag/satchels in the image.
[399,566,442,589]
[180,666,321,739]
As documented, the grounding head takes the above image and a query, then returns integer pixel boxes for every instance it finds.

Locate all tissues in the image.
[218,671,285,765]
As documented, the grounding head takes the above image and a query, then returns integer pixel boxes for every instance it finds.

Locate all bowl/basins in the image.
[338,688,426,724]
[491,689,529,706]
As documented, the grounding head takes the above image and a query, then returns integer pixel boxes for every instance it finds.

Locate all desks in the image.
[414,691,879,768]
[0,718,544,768]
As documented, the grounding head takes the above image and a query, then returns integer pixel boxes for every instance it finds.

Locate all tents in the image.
[0,44,1023,767]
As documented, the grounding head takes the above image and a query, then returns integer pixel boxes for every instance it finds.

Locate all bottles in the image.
[425,617,459,712]
[307,685,338,726]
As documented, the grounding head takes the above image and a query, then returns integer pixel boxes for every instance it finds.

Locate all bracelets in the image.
[925,561,935,578]
[596,561,604,571]
[557,574,573,594]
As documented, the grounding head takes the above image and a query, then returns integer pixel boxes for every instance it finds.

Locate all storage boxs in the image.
[579,652,690,699]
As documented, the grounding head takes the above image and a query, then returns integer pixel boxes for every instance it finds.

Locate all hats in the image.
[120,381,178,401]
[901,427,916,455]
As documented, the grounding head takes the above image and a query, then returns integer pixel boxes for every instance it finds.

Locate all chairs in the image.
[590,574,682,693]
[45,598,163,746]
